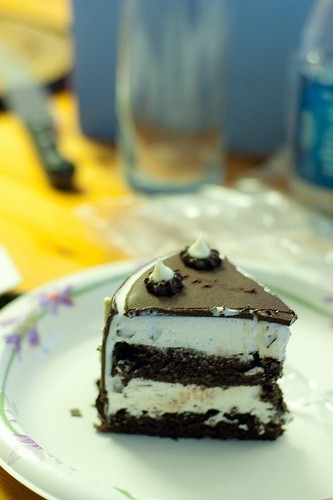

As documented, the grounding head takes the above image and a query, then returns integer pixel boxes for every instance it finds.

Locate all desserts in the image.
[95,234,298,441]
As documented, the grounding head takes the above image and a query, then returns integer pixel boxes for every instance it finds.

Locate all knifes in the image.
[2,45,78,191]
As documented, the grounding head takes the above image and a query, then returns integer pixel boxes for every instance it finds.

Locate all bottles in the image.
[288,0,332,215]
[115,0,224,198]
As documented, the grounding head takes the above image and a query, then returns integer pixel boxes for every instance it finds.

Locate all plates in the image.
[0,257,333,500]
